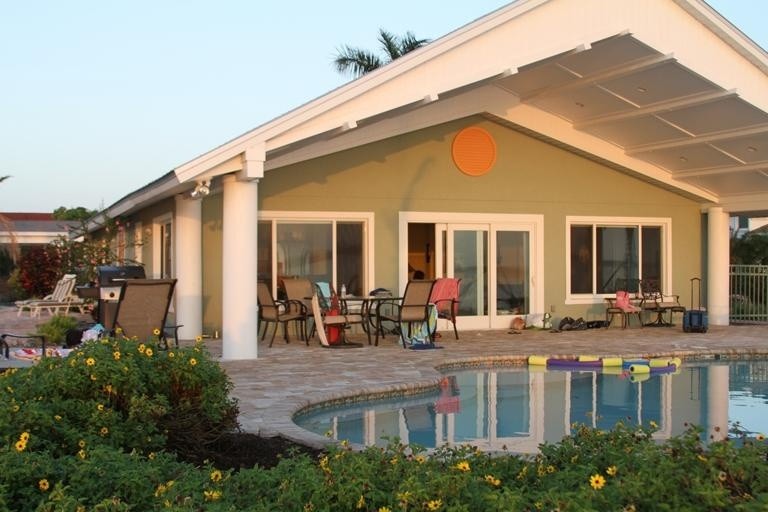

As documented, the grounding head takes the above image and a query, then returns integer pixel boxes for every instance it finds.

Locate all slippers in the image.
[508,330,521,334]
[550,329,562,333]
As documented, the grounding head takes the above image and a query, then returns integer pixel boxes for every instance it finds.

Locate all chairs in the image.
[258,275,463,350]
[605,277,681,328]
[0,274,184,377]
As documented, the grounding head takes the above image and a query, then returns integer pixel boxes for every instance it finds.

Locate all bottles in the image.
[341,283,346,299]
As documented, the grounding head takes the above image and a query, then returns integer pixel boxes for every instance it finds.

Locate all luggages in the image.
[683,278,708,333]
